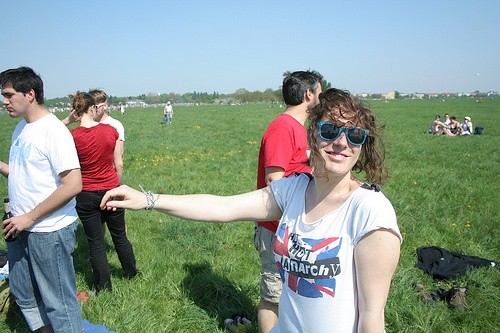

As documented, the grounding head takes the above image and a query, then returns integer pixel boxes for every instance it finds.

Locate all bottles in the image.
[3,198,17,242]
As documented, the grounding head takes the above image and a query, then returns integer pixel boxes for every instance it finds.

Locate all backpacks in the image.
[475,127,484,134]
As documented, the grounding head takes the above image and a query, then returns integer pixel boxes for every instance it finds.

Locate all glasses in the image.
[315,121,370,146]
[94,102,108,110]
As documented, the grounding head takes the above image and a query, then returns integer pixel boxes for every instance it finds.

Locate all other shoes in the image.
[88,282,113,297]
[423,284,468,312]
[223,314,255,333]
[123,271,144,280]
[166,122,171,125]
[446,131,454,136]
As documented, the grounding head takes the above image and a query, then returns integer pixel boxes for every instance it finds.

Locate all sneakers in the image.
[0,260,10,280]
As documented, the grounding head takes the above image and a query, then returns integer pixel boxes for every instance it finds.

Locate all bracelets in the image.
[28,211,37,222]
[139,184,155,210]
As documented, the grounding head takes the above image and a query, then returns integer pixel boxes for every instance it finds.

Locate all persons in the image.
[50,87,173,302]
[0,66,81,333]
[257,71,327,332]
[99,89,402,333]
[432,113,473,137]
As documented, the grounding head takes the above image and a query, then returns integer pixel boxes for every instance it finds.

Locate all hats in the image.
[166,100,171,105]
[464,116,472,122]
